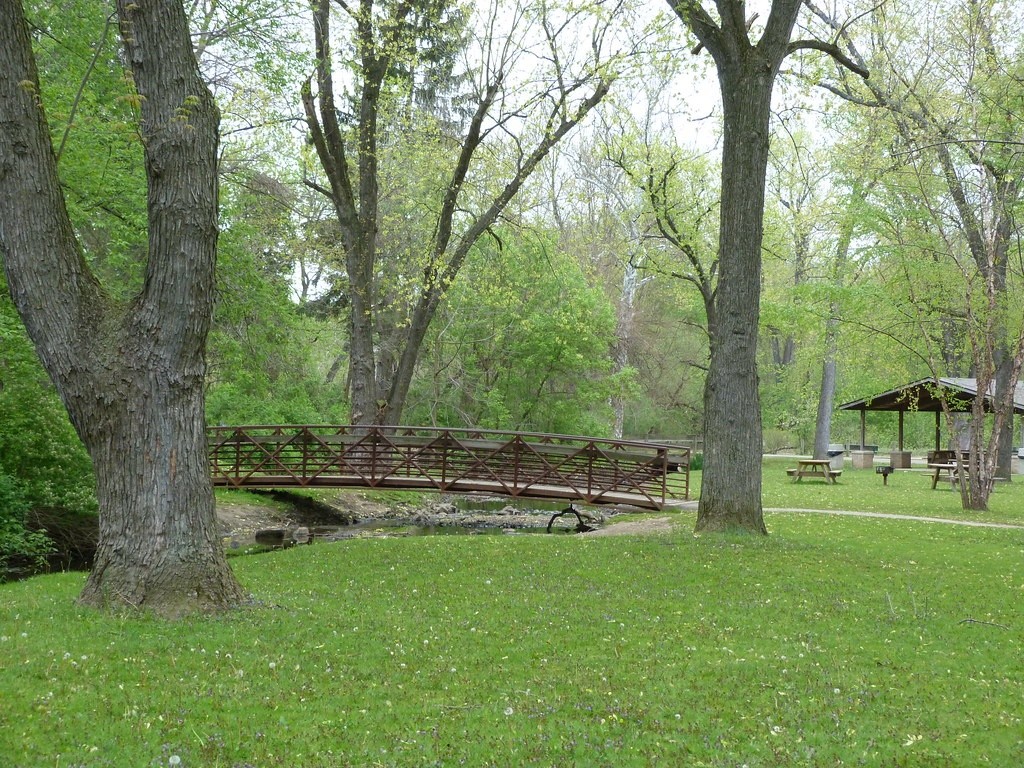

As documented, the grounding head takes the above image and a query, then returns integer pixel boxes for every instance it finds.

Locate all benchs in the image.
[787,469,796,473]
[922,474,1007,482]
[948,459,987,462]
[828,470,842,473]
[922,457,987,462]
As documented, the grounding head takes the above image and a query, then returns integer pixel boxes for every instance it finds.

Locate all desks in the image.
[792,460,837,485]
[928,450,956,468]
[929,463,1000,493]
[960,451,987,465]
